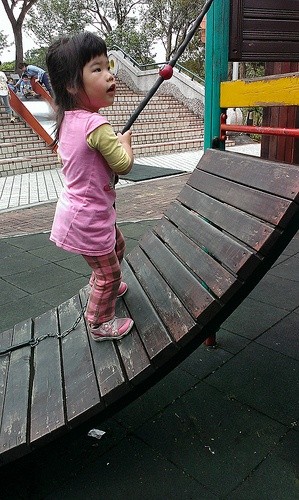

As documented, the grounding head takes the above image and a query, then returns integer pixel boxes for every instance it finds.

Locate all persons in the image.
[0,60,54,123]
[45,33,134,342]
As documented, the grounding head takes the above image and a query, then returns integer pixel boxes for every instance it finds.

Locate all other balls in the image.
[159,65,174,80]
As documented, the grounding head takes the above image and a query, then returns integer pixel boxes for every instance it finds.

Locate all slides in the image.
[6,75,60,152]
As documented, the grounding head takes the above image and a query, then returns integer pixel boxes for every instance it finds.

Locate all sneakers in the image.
[10,117,15,123]
[116,281,128,297]
[88,315,134,342]
